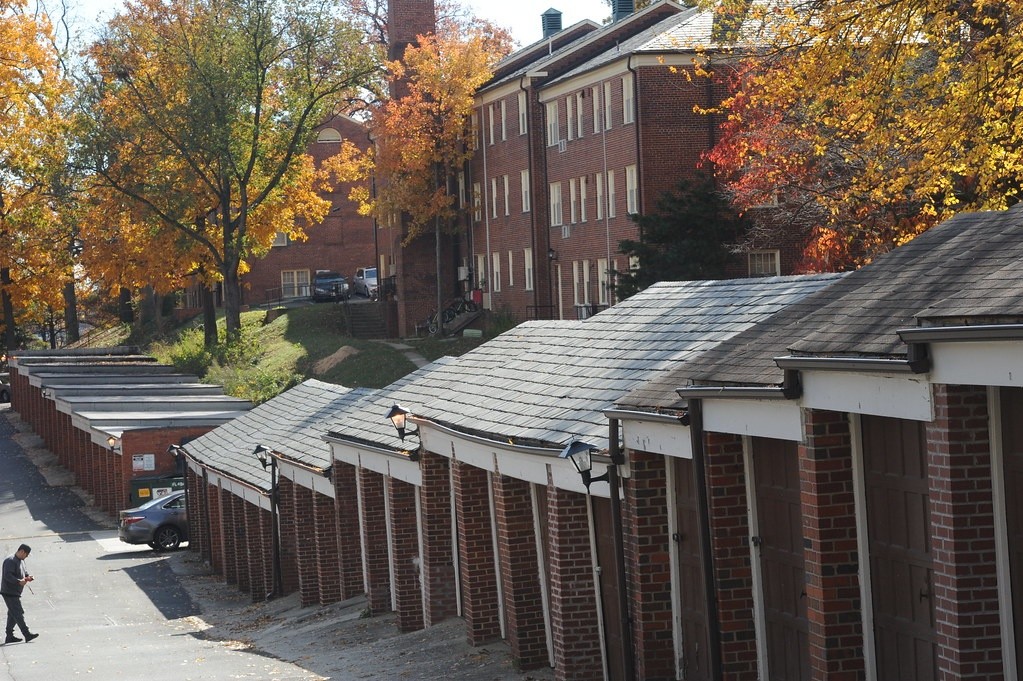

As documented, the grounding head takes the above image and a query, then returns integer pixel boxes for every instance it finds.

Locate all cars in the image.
[353,267,378,299]
[0,372,11,404]
[310,271,350,303]
[119,490,190,553]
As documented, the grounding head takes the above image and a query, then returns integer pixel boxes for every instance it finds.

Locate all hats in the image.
[22,544,31,552]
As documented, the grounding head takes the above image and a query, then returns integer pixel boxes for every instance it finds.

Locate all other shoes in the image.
[6,637,22,643]
[25,633,39,643]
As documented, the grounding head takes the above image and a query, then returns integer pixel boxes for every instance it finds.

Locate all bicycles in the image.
[425,293,476,334]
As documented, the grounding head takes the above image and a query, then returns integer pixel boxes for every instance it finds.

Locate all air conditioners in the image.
[577,304,593,320]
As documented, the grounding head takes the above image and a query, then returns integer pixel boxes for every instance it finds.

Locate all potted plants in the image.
[480,279,487,293]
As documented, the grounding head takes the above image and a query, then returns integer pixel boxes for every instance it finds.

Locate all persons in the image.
[0,544,39,643]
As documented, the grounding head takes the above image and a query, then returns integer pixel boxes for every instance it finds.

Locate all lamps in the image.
[39,384,51,399]
[252,445,272,472]
[547,248,557,260]
[106,436,120,454]
[384,401,418,443]
[557,435,609,495]
[165,444,182,467]
